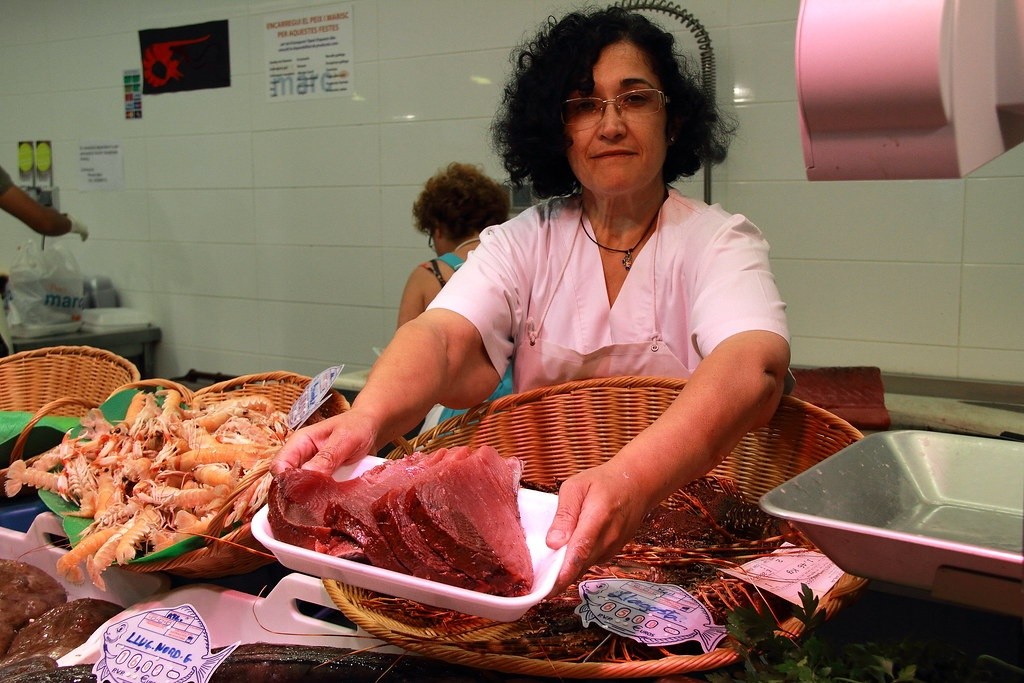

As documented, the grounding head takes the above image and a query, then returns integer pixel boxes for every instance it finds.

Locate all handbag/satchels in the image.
[5,240,86,340]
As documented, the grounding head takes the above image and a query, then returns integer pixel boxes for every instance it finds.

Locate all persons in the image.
[392,163,515,441]
[0,167,89,243]
[269,8,795,602]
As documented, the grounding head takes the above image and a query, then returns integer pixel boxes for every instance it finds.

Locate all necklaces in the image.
[579,200,661,269]
[453,238,481,251]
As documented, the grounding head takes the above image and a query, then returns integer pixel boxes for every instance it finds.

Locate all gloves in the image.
[68,215,89,241]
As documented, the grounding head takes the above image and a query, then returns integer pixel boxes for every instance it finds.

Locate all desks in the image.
[9,319,162,381]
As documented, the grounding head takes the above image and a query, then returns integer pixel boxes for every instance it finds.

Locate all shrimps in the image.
[4,386,293,592]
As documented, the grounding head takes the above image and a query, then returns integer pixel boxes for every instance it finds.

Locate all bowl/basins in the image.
[250,456,565,620]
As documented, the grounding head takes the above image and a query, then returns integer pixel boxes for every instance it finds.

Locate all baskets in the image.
[0,347,141,500]
[52,371,351,578]
[322,376,870,678]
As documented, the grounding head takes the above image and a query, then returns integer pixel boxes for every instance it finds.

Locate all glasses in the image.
[559,89,671,126]
[427,223,437,251]
